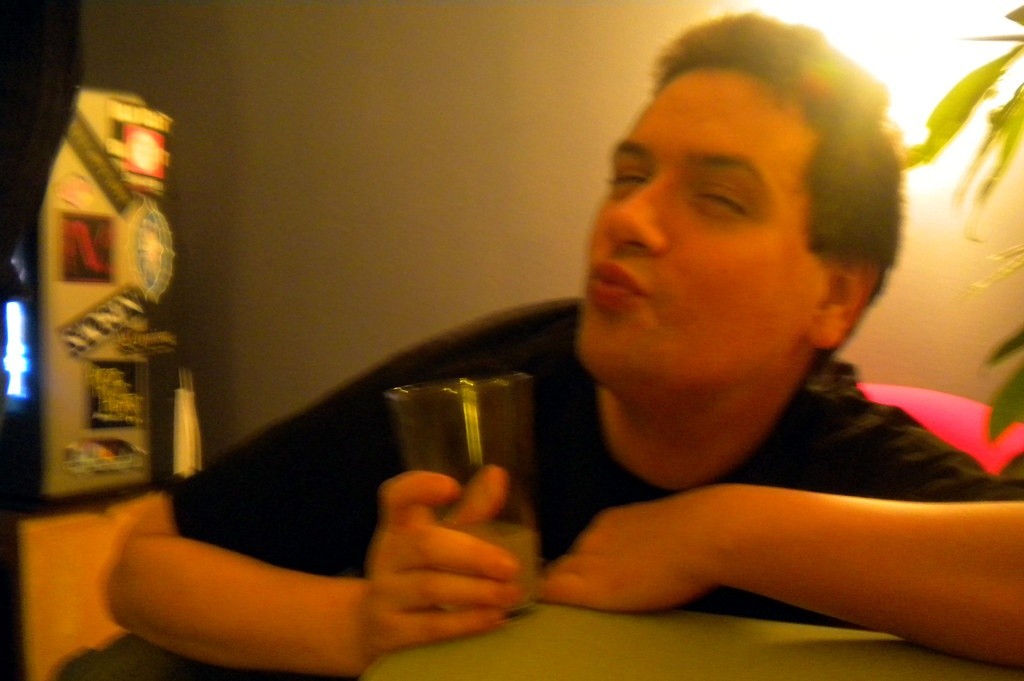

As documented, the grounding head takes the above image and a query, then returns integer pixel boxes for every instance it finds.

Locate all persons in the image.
[53,14,1024,681]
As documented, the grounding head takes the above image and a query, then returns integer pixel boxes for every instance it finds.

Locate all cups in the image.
[384,372,542,613]
